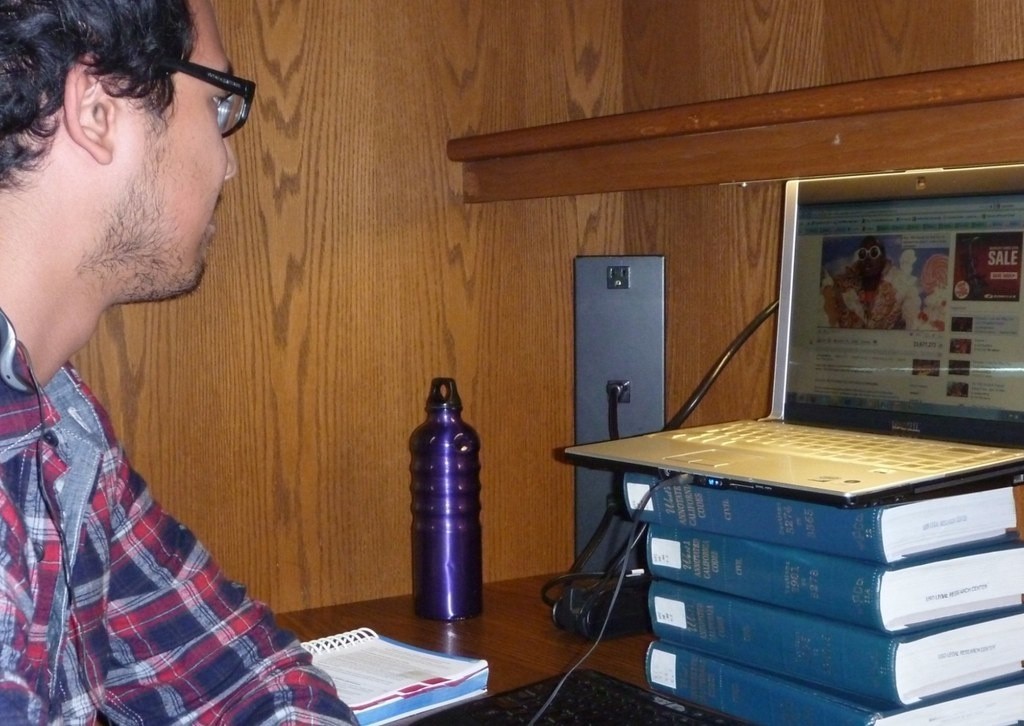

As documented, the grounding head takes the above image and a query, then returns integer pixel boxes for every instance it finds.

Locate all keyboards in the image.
[407,666,763,726]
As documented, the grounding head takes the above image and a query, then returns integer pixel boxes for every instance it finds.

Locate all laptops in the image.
[562,165,1024,510]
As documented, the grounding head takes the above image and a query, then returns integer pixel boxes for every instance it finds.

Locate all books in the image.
[623,468,1024,726]
[300,627,489,725]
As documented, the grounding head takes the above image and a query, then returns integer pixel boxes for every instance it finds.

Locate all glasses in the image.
[852,246,883,262]
[160,53,255,136]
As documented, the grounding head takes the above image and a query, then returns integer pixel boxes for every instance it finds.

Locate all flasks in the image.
[409,376,483,622]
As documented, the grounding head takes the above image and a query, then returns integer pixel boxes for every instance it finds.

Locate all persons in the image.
[1,0,357,726]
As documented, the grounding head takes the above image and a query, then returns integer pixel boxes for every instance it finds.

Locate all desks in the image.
[274,570,649,726]
